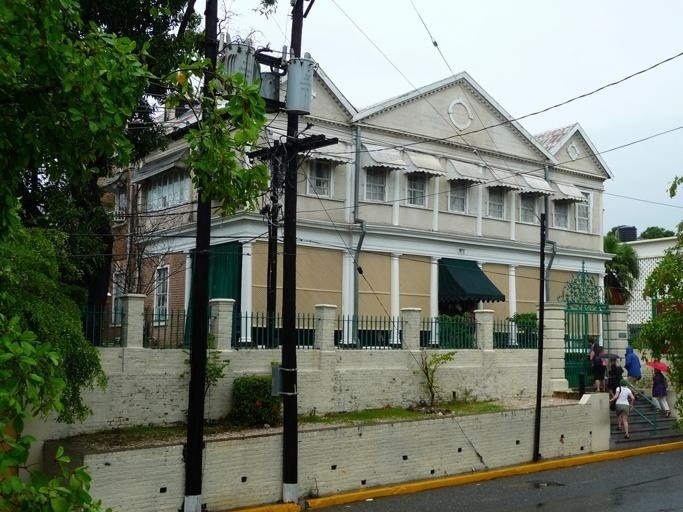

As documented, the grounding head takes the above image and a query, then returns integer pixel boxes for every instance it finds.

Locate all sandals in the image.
[616,426,622,430]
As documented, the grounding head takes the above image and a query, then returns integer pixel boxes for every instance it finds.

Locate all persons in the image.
[609,379,635,438]
[587,338,601,386]
[603,359,624,405]
[589,342,608,392]
[651,367,671,418]
[623,345,642,400]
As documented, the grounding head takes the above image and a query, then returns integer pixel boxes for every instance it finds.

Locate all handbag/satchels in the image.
[599,352,608,367]
[611,401,616,411]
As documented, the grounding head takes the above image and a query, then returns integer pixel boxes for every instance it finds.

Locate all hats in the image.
[619,378,627,385]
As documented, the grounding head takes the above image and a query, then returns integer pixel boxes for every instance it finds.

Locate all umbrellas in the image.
[645,360,669,373]
[599,352,620,360]
